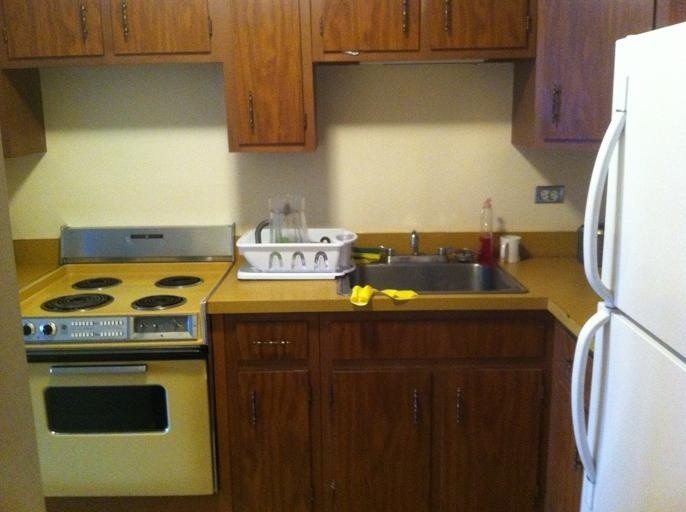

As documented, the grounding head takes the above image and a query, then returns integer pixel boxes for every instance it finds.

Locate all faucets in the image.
[411,229,420,255]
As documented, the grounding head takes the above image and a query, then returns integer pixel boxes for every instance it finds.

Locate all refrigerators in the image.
[572,20,684,512]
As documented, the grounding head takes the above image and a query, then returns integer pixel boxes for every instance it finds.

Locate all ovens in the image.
[23,354,220,498]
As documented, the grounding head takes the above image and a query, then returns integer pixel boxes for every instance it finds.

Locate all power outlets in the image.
[533,183,566,204]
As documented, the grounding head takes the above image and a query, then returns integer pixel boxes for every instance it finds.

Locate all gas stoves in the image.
[16,259,234,350]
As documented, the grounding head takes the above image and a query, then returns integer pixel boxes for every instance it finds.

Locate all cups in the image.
[254,192,311,244]
[498,234,522,263]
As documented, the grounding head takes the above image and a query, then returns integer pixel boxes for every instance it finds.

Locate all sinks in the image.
[335,259,529,297]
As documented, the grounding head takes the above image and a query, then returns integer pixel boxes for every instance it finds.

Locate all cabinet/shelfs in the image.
[652,0,686,31]
[209,308,319,512]
[317,310,545,512]
[509,1,657,151]
[0,0,216,67]
[215,0,317,154]
[312,0,537,63]
[0,62,48,162]
[545,312,593,512]
